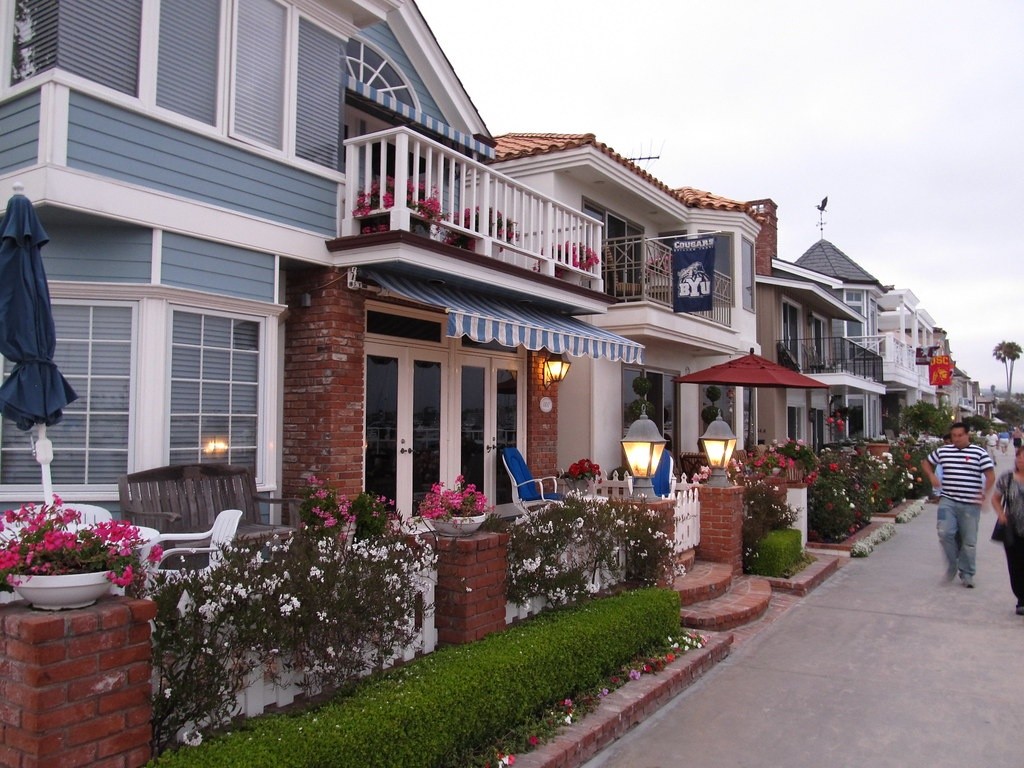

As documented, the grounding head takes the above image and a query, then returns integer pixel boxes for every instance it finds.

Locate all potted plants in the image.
[868,438,890,460]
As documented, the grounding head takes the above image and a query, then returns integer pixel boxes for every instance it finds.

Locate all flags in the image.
[672,236,717,313]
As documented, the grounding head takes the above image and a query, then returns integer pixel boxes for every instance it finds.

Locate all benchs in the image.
[119,464,305,568]
[680,450,748,483]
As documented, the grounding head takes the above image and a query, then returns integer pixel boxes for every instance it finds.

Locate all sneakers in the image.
[945,564,958,581]
[960,573,975,588]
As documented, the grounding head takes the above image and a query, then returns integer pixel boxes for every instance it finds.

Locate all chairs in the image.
[803,344,826,373]
[777,343,800,372]
[603,246,642,296]
[652,449,674,498]
[0,503,243,587]
[502,447,566,516]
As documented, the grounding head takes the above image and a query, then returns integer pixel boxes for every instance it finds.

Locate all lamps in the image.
[620,404,670,503]
[543,352,572,389]
[699,409,740,489]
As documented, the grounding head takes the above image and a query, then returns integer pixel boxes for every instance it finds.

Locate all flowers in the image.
[417,474,496,522]
[691,437,820,487]
[343,176,521,252]
[0,494,163,599]
[304,475,399,549]
[560,459,602,484]
[532,241,599,278]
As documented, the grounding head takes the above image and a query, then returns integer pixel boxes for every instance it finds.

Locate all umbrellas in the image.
[671,348,831,454]
[0,182,78,507]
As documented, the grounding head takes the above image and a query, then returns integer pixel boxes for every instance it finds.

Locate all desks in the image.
[0,524,159,549]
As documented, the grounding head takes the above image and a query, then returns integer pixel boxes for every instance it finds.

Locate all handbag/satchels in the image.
[992,472,1012,540]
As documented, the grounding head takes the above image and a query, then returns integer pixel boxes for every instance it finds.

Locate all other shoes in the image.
[1016,606,1024,614]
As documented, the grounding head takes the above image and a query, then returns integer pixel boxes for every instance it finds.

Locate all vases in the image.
[341,524,356,548]
[573,480,587,496]
[9,570,112,610]
[429,513,486,536]
[562,271,584,287]
[410,216,430,239]
[461,237,475,252]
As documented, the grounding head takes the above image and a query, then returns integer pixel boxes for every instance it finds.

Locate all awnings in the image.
[367,269,646,366]
[344,73,495,159]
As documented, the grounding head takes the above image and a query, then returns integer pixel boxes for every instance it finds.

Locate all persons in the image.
[985,425,1024,465]
[992,448,1024,614]
[922,422,995,588]
